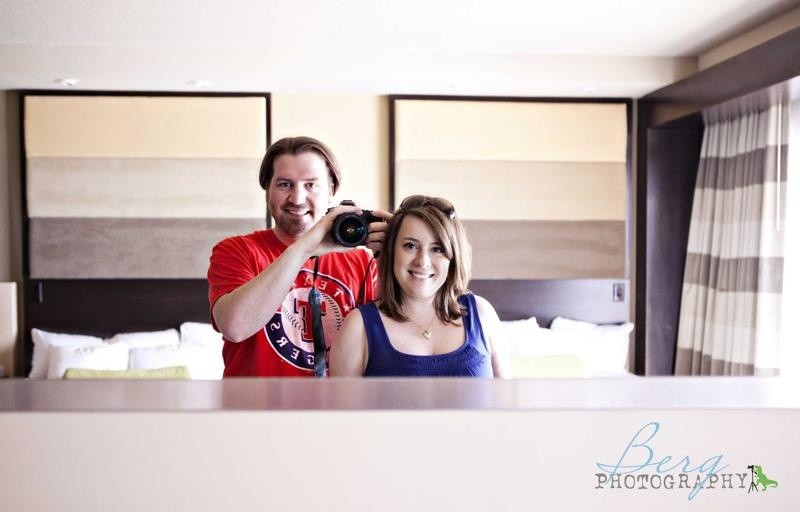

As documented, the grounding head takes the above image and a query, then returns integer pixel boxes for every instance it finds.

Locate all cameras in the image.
[325,199,384,249]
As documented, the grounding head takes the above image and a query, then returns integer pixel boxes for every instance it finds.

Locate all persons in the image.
[329,196,526,377]
[207,136,394,377]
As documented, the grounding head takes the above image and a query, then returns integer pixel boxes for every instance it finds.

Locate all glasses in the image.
[398,195,455,218]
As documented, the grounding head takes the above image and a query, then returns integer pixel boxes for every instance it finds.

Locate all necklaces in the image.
[410,314,436,340]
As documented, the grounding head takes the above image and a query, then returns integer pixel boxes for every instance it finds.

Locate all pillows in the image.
[28,320,224,379]
[484,314,635,378]
